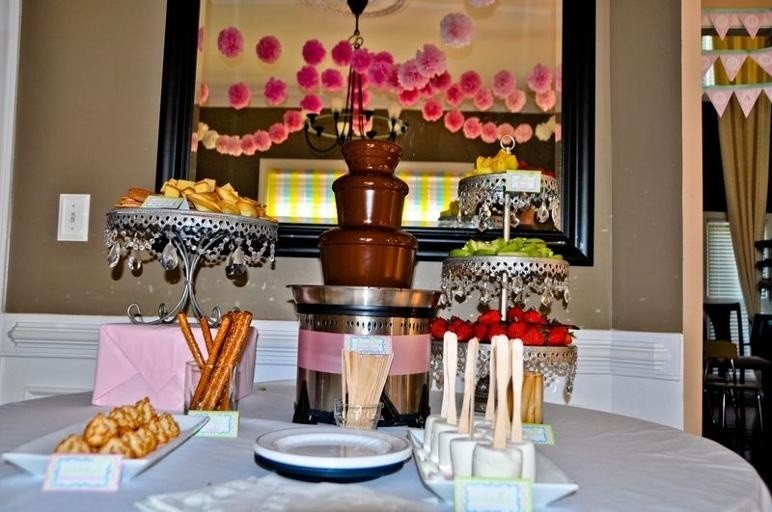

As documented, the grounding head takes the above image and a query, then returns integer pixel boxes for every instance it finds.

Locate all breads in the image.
[55,396,180,459]
[115,177,279,224]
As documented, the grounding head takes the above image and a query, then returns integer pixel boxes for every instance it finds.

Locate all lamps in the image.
[304,0,409,152]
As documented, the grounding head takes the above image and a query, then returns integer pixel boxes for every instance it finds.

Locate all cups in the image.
[177,360,238,412]
[333,400,384,432]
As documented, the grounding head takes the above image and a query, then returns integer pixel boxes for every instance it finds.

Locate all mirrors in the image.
[155,0,596,265]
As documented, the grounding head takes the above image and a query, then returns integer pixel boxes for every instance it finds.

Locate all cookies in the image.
[178,310,253,410]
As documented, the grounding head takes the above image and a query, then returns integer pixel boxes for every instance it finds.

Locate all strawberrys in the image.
[430,306,580,347]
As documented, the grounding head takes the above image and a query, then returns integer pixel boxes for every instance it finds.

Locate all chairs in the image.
[703,303,768,428]
[703,339,765,459]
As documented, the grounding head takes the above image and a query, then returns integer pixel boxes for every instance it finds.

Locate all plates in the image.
[6,406,207,484]
[411,429,577,511]
[252,429,415,482]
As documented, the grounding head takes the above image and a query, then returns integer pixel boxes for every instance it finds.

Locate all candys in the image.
[424,331,537,481]
